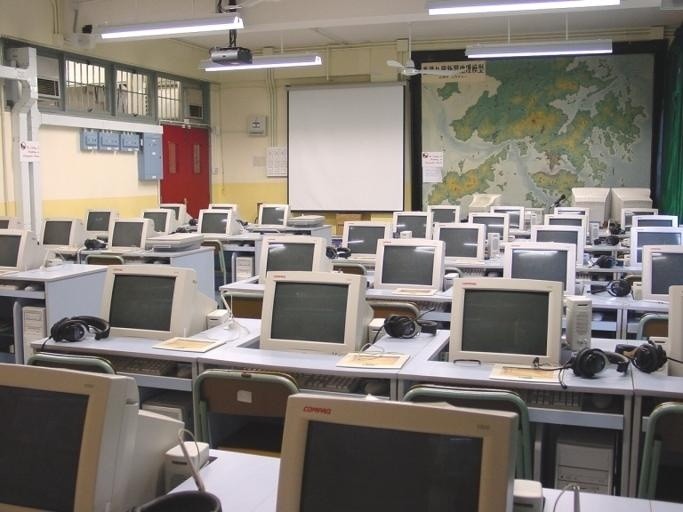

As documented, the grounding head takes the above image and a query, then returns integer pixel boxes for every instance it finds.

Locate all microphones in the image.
[548,194,567,209]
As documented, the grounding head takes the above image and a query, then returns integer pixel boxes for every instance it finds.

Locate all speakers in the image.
[399,230,413,238]
[565,298,592,350]
[45,258,64,267]
[444,272,459,290]
[633,281,642,301]
[205,308,231,330]
[487,232,501,257]
[529,213,539,226]
[589,222,599,244]
[368,316,388,345]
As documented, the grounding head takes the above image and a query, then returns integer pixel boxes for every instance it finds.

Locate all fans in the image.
[387,59,459,77]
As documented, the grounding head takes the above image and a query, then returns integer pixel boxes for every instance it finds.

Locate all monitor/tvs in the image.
[628,226,682,268]
[258,270,375,355]
[341,220,393,259]
[392,210,433,238]
[0,228,57,276]
[489,204,525,232]
[432,222,487,260]
[373,238,446,292]
[468,211,510,244]
[99,263,219,342]
[569,186,611,228]
[502,241,576,296]
[258,234,327,285]
[107,218,159,249]
[528,224,584,267]
[207,203,238,214]
[0,363,184,512]
[158,203,194,226]
[197,209,241,236]
[543,214,588,234]
[278,395,517,512]
[552,207,591,217]
[447,276,564,366]
[0,217,28,228]
[426,205,461,222]
[631,215,679,227]
[39,218,84,248]
[610,187,654,223]
[139,208,179,236]
[609,222,625,234]
[670,284,683,378]
[641,244,683,302]
[81,209,121,237]
[258,202,293,226]
[469,193,501,213]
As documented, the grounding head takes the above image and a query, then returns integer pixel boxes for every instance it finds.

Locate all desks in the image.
[333,253,618,282]
[185,223,332,247]
[0,263,111,365]
[197,318,441,449]
[219,271,622,340]
[586,228,631,242]
[397,329,634,497]
[46,246,216,303]
[617,266,643,280]
[165,449,683,512]
[622,290,670,341]
[29,317,260,403]
[627,339,683,498]
[499,238,630,257]
[200,233,264,284]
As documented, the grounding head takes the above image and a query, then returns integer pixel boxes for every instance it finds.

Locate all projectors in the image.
[209,46,253,67]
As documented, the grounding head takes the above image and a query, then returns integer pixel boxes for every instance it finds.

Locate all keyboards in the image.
[106,355,177,377]
[0,280,30,292]
[511,387,583,411]
[413,300,447,314]
[226,365,361,394]
[220,240,242,247]
[460,267,486,278]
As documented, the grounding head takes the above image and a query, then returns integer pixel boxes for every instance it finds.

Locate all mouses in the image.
[23,283,44,292]
[593,394,616,410]
[488,272,499,277]
[295,232,302,235]
[364,380,389,395]
[152,259,167,264]
[591,310,605,322]
[243,242,251,247]
[178,364,193,378]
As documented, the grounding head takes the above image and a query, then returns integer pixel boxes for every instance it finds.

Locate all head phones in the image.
[84,238,107,250]
[383,315,438,339]
[188,218,198,225]
[49,315,111,343]
[175,226,197,234]
[586,279,632,297]
[589,255,615,269]
[593,235,620,245]
[325,246,353,260]
[569,345,630,379]
[615,342,670,375]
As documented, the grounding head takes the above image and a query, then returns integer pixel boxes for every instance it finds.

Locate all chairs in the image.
[366,300,419,322]
[638,402,683,505]
[201,240,226,286]
[85,254,125,265]
[400,384,533,480]
[445,268,463,278]
[636,313,669,340]
[193,369,301,457]
[331,263,367,276]
[218,292,264,320]
[26,352,118,374]
[623,275,641,286]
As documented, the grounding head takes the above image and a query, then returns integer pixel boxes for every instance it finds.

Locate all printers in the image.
[144,233,205,252]
[288,214,326,227]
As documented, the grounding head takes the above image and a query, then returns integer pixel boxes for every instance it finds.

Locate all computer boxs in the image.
[22,307,48,367]
[554,437,616,495]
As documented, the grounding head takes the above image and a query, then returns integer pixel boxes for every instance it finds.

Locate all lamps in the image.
[428,0,619,17]
[100,16,244,40]
[198,55,322,72]
[464,39,613,59]
